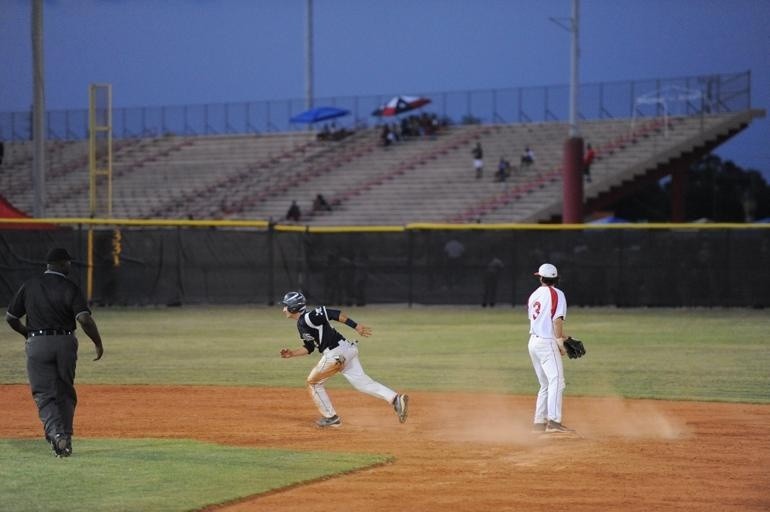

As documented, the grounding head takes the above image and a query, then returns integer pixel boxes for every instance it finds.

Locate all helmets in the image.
[281,291,306,314]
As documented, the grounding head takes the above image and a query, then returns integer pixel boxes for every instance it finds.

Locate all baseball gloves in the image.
[564,338,585,359]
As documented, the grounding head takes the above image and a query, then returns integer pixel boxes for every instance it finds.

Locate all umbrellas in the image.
[289,106,349,122]
[370,95,430,116]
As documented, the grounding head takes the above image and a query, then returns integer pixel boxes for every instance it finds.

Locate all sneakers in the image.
[393,394,409,423]
[316,416,341,427]
[532,420,568,434]
[52,434,72,457]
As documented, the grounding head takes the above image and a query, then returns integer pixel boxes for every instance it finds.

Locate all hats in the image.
[46,248,76,262]
[533,263,558,278]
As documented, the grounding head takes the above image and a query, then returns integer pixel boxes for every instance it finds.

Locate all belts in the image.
[27,329,72,336]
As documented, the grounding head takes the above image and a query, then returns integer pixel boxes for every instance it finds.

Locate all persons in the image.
[495,146,536,181]
[6,248,104,457]
[315,123,336,139]
[280,292,409,428]
[471,143,483,178]
[285,199,299,222]
[583,143,595,184]
[379,113,439,145]
[526,263,575,432]
[313,194,330,211]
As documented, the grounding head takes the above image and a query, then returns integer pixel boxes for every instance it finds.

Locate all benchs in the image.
[0,110,764,225]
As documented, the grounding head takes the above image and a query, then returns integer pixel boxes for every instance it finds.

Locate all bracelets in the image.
[554,336,564,347]
[343,317,358,329]
